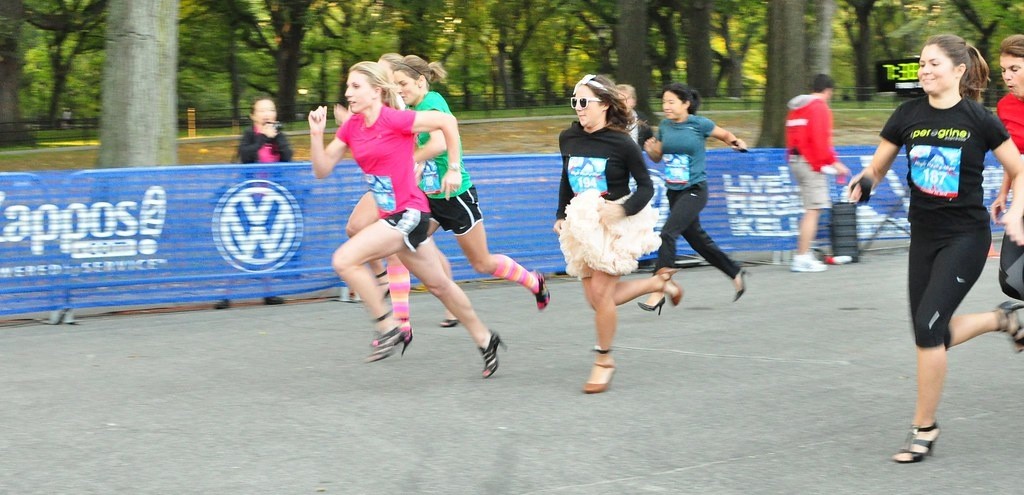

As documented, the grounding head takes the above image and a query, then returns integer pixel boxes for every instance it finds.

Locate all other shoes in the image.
[264,296,285,304]
[214,300,229,308]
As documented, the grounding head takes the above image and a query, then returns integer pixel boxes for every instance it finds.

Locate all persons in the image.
[552,74,686,394]
[345,53,550,359]
[637,83,751,315]
[847,35,1024,464]
[784,74,851,272]
[990,34,1024,300]
[308,61,507,379]
[613,84,654,151]
[213,97,292,310]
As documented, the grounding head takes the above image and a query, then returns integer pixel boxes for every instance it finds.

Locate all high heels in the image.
[733,268,752,302]
[993,300,1024,353]
[478,330,508,378]
[581,362,615,392]
[638,298,665,315]
[892,422,938,463]
[657,267,685,306]
[365,328,411,362]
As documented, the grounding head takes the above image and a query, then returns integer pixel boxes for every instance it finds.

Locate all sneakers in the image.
[532,271,550,311]
[791,253,827,273]
[372,325,413,346]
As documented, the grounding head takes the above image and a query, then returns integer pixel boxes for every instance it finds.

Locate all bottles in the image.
[825,255,853,265]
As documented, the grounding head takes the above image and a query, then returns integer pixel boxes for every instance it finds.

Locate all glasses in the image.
[569,96,604,109]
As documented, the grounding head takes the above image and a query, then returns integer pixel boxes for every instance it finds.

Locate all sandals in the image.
[440,318,459,327]
[375,270,393,299]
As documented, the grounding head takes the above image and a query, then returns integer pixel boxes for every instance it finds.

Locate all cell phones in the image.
[731,141,748,153]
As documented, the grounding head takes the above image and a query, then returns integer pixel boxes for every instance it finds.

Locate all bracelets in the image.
[446,163,460,171]
